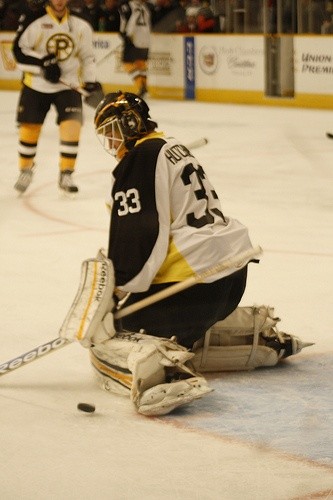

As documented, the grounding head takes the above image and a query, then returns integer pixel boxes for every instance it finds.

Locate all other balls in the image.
[78,403,96,412]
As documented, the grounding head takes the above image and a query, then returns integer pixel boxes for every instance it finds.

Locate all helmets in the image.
[94,91,149,155]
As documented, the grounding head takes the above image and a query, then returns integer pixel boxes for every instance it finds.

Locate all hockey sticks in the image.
[59,78,207,150]
[0,246,262,376]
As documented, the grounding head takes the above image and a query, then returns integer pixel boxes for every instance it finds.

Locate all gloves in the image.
[83,81,103,106]
[41,55,60,83]
[78,297,115,348]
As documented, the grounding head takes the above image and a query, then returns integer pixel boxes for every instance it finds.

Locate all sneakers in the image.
[14,170,33,198]
[278,331,317,358]
[59,170,78,199]
[140,372,215,417]
